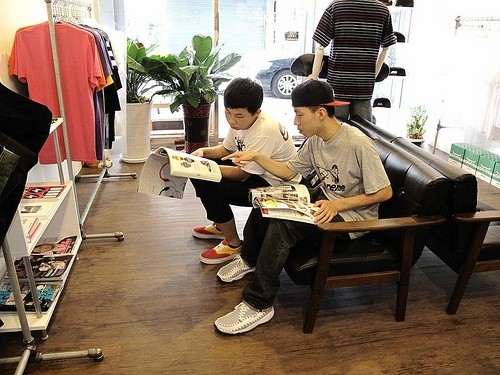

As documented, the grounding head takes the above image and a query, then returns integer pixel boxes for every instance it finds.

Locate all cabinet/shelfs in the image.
[0,111,84,342]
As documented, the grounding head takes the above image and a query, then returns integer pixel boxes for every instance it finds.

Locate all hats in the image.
[291,78,350,107]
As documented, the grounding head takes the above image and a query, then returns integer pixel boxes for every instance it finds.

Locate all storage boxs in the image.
[450,142,500,189]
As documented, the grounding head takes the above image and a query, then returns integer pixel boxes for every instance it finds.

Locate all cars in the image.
[255,58,298,99]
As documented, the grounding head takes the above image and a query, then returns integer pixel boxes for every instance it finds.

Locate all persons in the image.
[310,0,396,119]
[190,78,296,265]
[33,259,67,272]
[32,236,77,253]
[214,78,392,335]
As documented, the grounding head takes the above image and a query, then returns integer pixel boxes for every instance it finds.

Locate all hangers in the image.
[46,0,94,25]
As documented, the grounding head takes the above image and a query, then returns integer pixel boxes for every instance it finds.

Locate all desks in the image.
[352,114,500,315]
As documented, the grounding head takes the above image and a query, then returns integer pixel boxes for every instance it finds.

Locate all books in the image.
[136,147,221,199]
[249,184,323,225]
[0,185,79,313]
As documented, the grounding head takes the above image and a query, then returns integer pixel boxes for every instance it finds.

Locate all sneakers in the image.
[217,252,256,283]
[199,237,244,264]
[214,301,274,334]
[192,223,226,239]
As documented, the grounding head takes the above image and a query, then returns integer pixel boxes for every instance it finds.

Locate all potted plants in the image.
[121,36,170,166]
[404,103,429,147]
[140,31,242,153]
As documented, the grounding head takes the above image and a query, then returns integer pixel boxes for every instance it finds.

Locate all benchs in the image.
[265,113,451,333]
